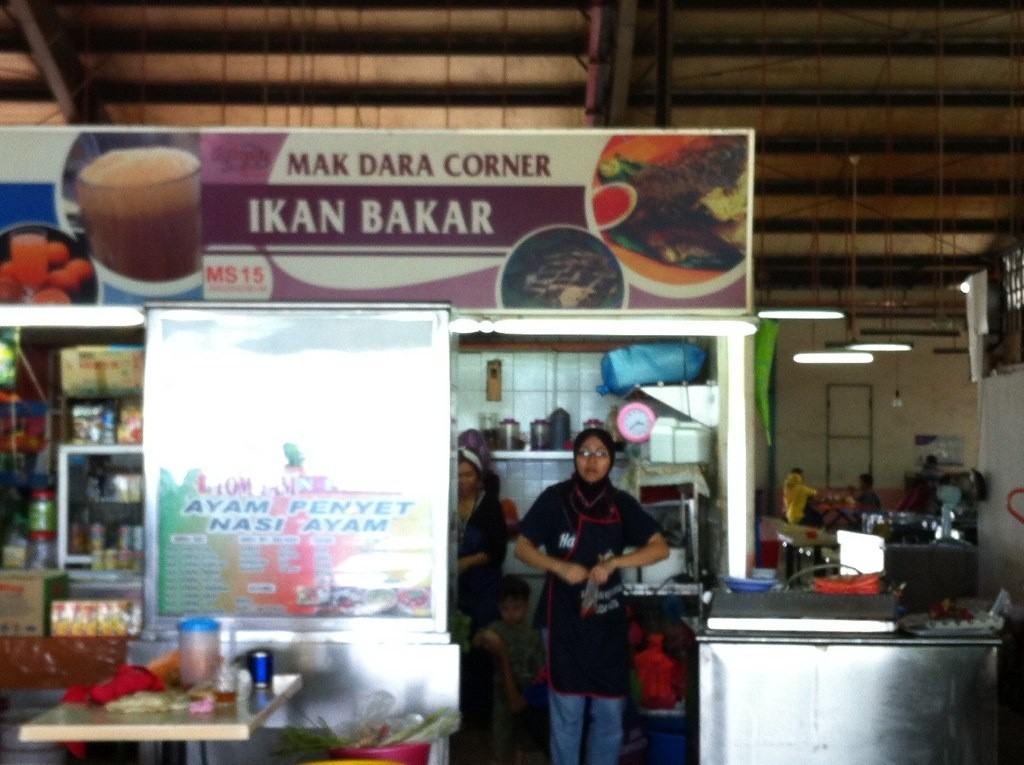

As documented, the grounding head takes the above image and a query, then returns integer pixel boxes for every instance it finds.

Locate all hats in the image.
[458,446,483,473]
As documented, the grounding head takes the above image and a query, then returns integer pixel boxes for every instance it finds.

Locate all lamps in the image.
[844,310,914,352]
[931,337,969,355]
[858,318,961,337]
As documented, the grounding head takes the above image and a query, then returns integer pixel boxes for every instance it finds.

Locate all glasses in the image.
[576,451,608,459]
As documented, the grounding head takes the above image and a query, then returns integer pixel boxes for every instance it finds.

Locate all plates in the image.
[586,135,747,299]
[720,573,775,593]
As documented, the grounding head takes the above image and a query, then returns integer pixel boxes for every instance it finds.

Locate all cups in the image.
[530,419,548,450]
[78,149,202,284]
[500,418,519,451]
[247,650,273,689]
[177,618,220,691]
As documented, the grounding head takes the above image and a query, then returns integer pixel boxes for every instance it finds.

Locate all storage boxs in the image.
[0,569,69,639]
[58,343,144,399]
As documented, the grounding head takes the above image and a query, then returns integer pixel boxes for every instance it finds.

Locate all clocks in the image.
[617,401,656,443]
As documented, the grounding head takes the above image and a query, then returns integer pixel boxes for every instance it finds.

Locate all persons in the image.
[783,468,881,527]
[445,430,546,765]
[512,427,669,765]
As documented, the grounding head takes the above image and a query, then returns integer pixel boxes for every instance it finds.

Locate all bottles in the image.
[70,504,142,554]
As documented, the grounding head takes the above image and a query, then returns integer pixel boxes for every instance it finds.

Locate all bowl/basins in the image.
[495,225,629,310]
[0,223,103,306]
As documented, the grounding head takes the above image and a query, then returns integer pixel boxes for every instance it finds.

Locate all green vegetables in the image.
[267,720,346,762]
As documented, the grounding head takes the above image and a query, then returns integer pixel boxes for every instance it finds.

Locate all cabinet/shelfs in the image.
[55,444,145,581]
[620,458,711,717]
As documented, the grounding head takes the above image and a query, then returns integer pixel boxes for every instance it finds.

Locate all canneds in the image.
[69,518,143,572]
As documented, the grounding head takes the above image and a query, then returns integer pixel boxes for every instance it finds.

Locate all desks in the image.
[18,674,303,765]
[820,504,862,530]
